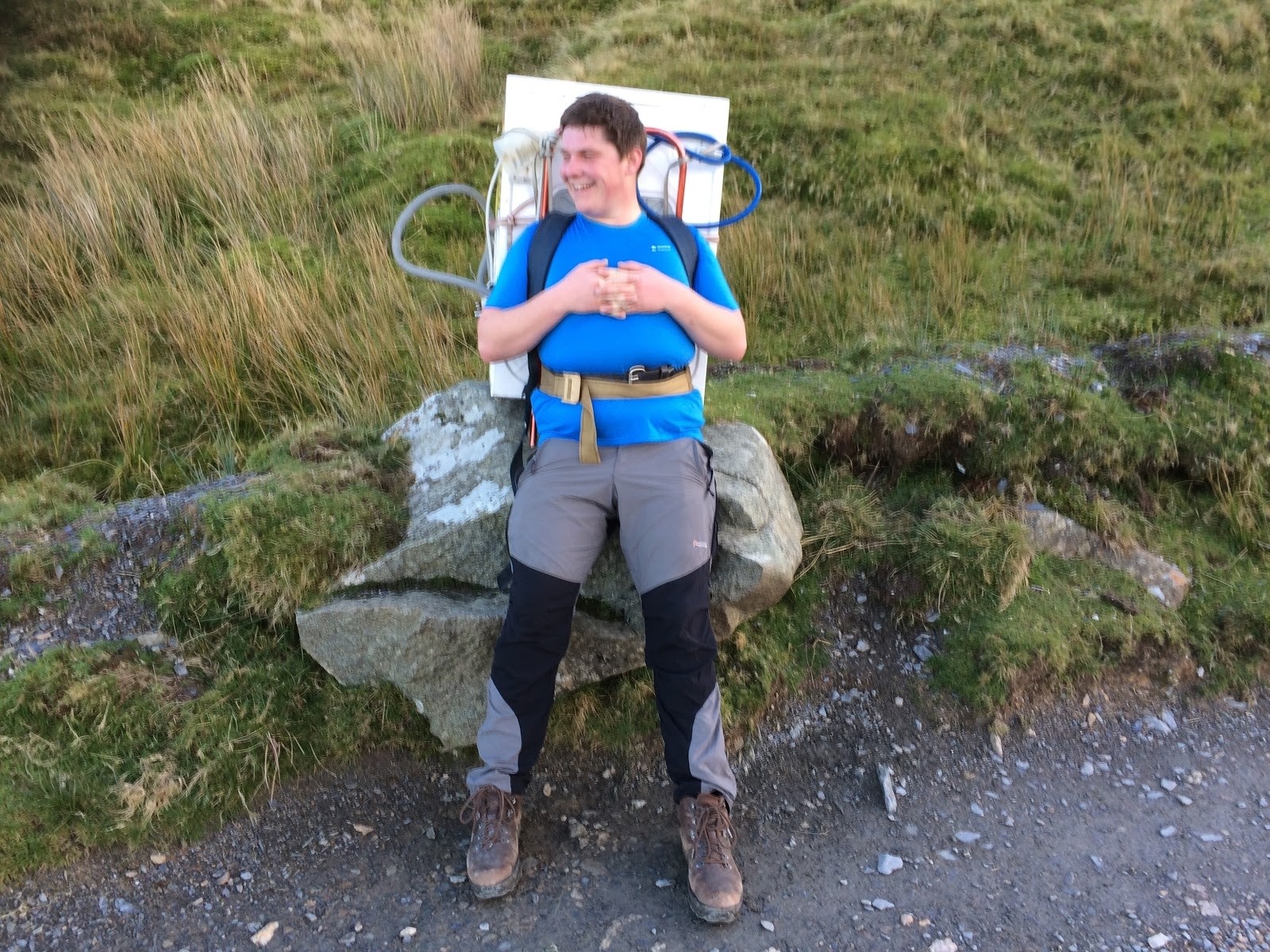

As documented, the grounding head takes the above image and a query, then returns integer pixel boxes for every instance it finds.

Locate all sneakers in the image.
[676,794,744,923]
[459,784,523,900]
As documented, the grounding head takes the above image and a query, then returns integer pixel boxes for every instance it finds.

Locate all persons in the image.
[458,97,750,926]
